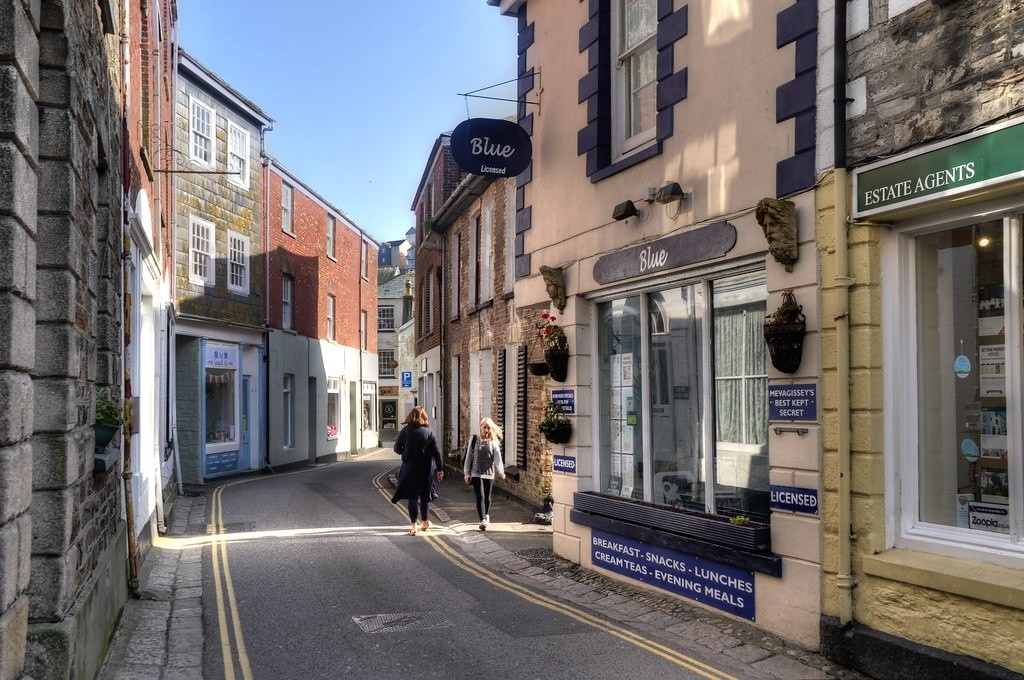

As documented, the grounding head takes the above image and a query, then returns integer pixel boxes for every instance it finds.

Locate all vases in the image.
[544,346,570,382]
[528,362,549,376]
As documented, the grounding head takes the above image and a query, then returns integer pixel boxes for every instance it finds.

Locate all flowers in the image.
[537,313,566,351]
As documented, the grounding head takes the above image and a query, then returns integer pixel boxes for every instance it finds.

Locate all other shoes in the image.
[485,514,490,525]
[479,520,487,531]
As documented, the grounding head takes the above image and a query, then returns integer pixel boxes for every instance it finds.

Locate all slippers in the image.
[410,529,418,535]
[420,523,430,530]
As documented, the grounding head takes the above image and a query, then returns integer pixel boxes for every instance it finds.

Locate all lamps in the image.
[656,183,687,206]
[422,242,442,252]
[612,200,640,221]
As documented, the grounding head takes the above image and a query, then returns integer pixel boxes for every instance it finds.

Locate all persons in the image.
[754,195,794,262]
[464,417,506,531]
[538,265,563,308]
[391,406,443,536]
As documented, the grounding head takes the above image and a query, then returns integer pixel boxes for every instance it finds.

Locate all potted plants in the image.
[93,389,124,453]
[539,400,572,445]
[763,289,807,374]
[574,489,771,552]
